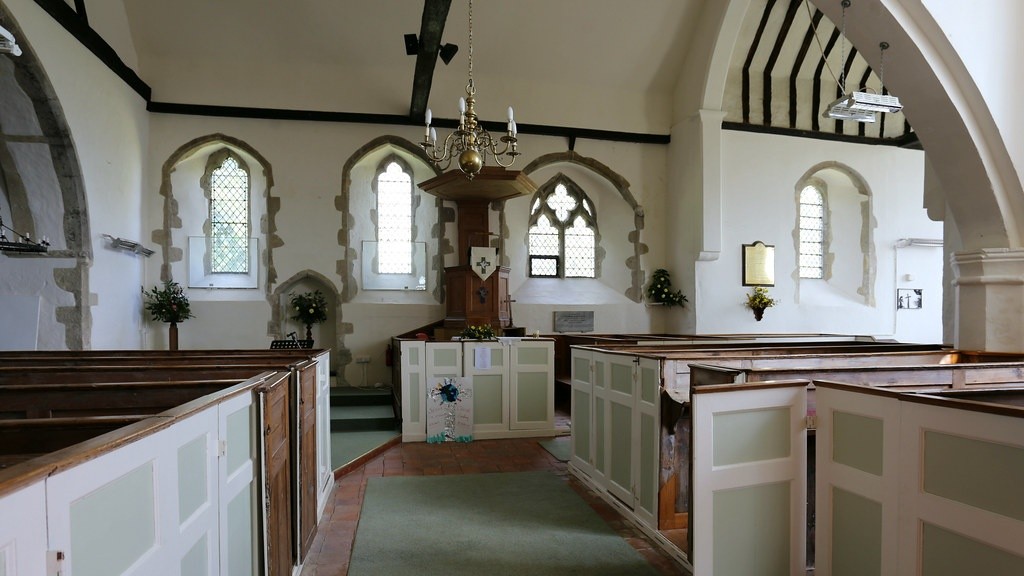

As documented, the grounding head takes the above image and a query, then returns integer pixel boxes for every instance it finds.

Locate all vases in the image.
[751,307,766,321]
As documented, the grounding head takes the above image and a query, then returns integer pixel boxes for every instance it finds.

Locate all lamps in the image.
[105,235,154,257]
[403,33,419,55]
[0,215,52,254]
[438,43,458,65]
[0,25,22,56]
[822,0,904,123]
[419,0,522,181]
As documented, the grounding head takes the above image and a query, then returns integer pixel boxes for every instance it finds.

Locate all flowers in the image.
[459,323,498,341]
[746,286,775,308]
[288,289,328,325]
[143,278,196,322]
[646,268,689,308]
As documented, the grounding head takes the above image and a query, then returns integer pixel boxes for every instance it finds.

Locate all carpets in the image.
[347,469,660,576]
[537,440,573,461]
